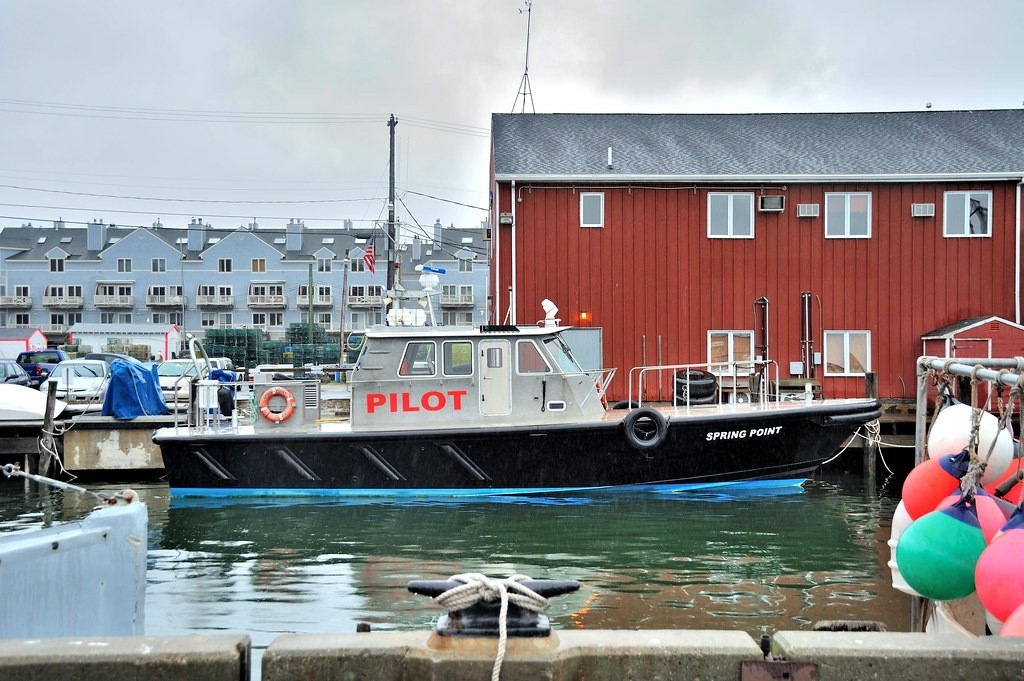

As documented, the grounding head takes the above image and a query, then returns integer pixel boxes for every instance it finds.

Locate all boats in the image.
[0,490,149,639]
[912,357,1024,634]
[152,261,883,496]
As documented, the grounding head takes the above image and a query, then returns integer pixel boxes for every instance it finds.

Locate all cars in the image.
[39,353,256,413]
[0,359,32,387]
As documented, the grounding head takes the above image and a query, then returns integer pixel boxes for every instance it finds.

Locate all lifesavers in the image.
[670,390,716,407]
[671,369,716,398]
[612,400,644,409]
[623,407,668,451]
[258,386,296,422]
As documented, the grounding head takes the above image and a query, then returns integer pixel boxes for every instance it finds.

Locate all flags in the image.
[363,238,376,276]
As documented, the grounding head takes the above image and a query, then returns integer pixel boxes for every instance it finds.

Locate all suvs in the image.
[17,351,75,390]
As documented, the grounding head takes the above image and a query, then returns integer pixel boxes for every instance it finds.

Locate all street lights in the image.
[386,202,396,327]
